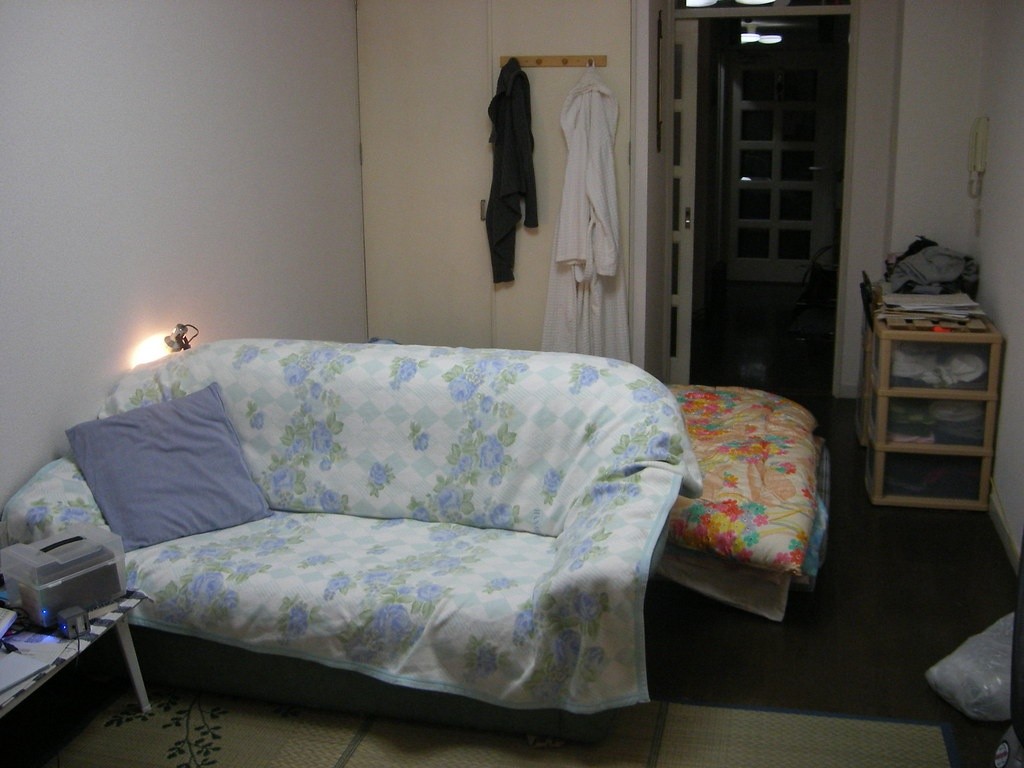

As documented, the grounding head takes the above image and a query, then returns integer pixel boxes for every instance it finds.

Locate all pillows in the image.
[66,382,272,554]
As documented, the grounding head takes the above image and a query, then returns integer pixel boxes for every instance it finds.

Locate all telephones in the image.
[967,117,987,173]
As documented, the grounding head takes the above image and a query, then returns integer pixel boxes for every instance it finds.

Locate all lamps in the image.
[164,323,200,351]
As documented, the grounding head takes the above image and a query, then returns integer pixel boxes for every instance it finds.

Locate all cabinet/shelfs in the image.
[853,282,1003,511]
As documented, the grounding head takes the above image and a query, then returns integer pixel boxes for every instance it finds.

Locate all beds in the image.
[668,382,833,623]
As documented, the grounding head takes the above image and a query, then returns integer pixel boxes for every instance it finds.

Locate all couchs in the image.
[2,339,705,722]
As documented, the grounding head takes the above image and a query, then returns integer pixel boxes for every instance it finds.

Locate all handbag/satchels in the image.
[795,244,841,309]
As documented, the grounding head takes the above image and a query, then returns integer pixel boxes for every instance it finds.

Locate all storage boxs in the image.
[1,521,126,626]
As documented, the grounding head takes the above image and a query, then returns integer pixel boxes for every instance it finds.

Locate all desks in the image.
[0,587,155,721]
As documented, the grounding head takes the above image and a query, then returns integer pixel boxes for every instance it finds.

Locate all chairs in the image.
[860,270,875,332]
